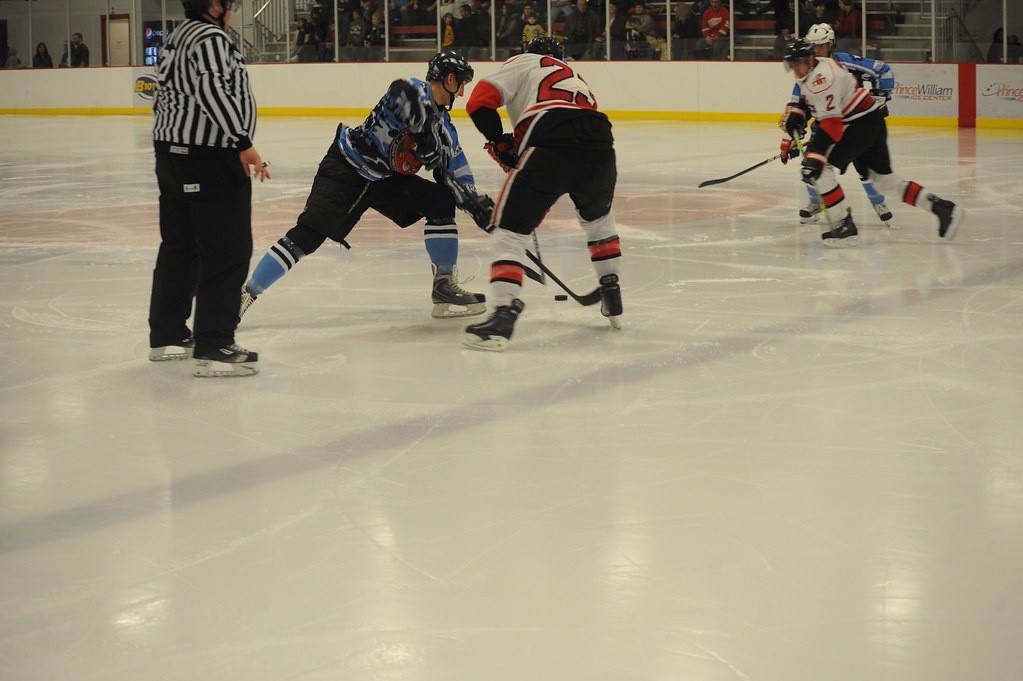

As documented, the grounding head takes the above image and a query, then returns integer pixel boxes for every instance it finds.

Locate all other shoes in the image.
[290,55,300,62]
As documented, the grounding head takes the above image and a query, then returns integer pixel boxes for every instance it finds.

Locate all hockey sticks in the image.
[792,129,852,225]
[698,139,813,188]
[508,146,547,286]
[411,141,602,307]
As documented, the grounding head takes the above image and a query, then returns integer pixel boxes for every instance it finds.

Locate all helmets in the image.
[784,38,814,62]
[527,37,564,61]
[426,49,473,83]
[806,23,835,48]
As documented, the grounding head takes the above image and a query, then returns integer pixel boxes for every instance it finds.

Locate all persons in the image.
[671,0,751,60]
[466,34,624,340]
[778,23,955,240]
[987,28,1023,64]
[495,0,665,61]
[756,0,870,60]
[72,33,89,68]
[390,0,492,61]
[5,47,22,68]
[32,42,55,68]
[237,49,496,326]
[58,40,75,67]
[148,0,271,365]
[285,0,385,63]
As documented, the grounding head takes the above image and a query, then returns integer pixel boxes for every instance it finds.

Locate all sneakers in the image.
[194,340,259,376]
[799,204,821,224]
[148,332,194,361]
[928,193,964,241]
[821,208,858,247]
[462,298,524,351]
[430,263,487,317]
[873,201,893,227]
[238,286,257,324]
[599,273,623,329]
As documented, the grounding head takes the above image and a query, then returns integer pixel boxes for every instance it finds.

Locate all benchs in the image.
[257,0,958,61]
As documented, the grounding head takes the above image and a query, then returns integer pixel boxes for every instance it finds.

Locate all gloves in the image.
[485,132,518,173]
[786,104,808,139]
[468,194,496,233]
[869,88,891,116]
[800,146,827,183]
[780,132,799,164]
[409,117,443,171]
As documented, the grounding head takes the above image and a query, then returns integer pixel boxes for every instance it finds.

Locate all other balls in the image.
[554,294,568,300]
